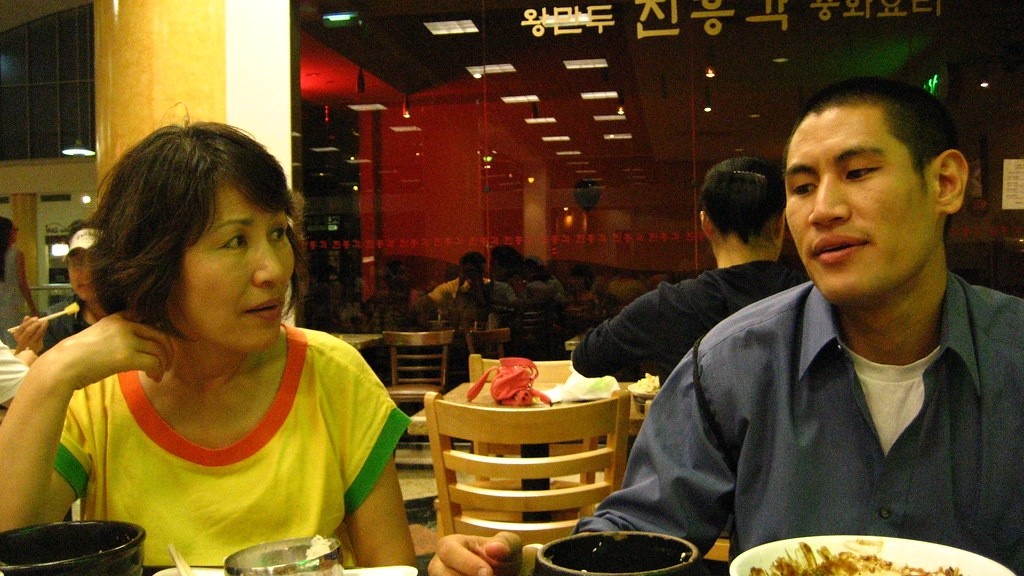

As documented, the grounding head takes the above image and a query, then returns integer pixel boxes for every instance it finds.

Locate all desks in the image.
[328,333,384,352]
[404,380,643,436]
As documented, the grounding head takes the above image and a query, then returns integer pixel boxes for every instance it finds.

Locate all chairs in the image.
[358,302,630,557]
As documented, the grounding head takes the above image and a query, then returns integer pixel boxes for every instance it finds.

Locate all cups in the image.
[224,536,344,576]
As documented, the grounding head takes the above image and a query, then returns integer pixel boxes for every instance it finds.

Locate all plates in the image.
[152,564,419,576]
[731,535,1016,576]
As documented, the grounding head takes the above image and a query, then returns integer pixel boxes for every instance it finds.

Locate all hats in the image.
[62,228,104,263]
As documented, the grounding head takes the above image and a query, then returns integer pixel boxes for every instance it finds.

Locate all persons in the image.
[305,245,680,383]
[571,156,810,381]
[0,119,413,576]
[426,75,1024,576]
[0,214,109,423]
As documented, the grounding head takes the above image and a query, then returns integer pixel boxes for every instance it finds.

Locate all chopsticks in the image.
[7,310,66,333]
[170,547,193,576]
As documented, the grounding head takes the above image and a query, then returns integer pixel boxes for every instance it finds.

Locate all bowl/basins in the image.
[533,531,699,576]
[0,520,146,576]
